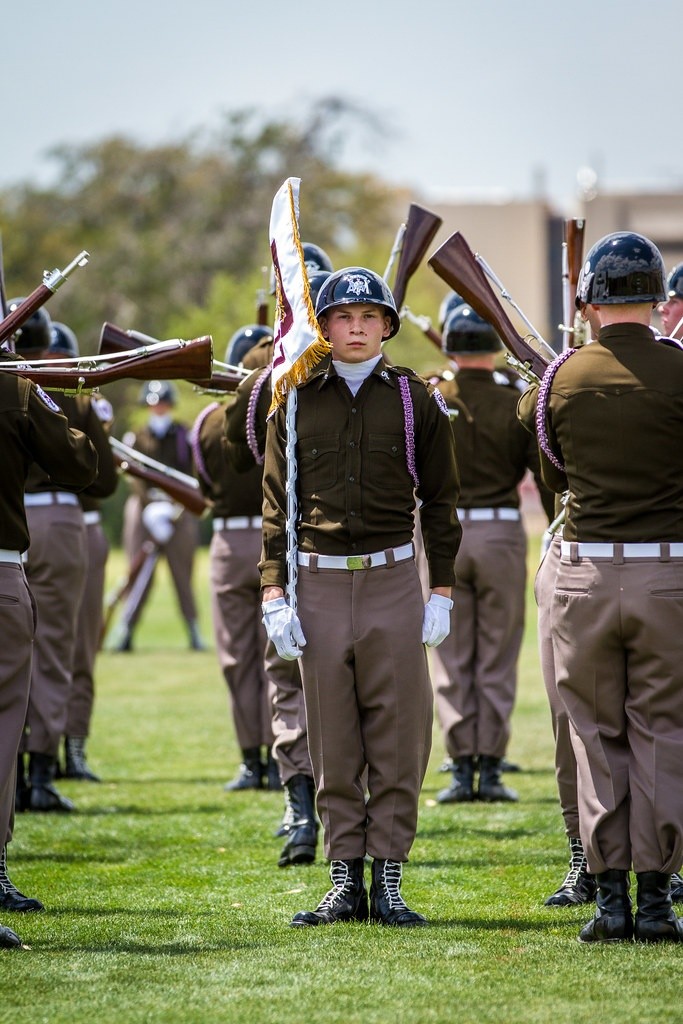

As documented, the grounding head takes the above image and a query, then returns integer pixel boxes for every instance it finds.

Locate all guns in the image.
[389,202,443,315]
[557,217,586,355]
[97,318,254,394]
[0,334,217,391]
[428,229,560,385]
[0,249,94,343]
[106,430,217,522]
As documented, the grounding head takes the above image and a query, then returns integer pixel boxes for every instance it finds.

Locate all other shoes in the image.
[0,923,22,948]
[438,759,519,773]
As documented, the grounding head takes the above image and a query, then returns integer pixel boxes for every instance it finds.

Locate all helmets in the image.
[4,240,683,352]
[139,379,177,407]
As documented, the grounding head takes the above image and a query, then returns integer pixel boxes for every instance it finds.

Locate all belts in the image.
[212,515,262,531]
[83,509,100,525]
[561,540,683,557]
[24,491,80,507]
[298,542,413,570]
[456,507,520,522]
[553,524,565,537]
[0,549,28,565]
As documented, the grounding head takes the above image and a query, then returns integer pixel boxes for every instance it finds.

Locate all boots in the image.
[578,870,633,944]
[367,857,429,927]
[545,837,597,908]
[669,872,683,903]
[437,754,521,803]
[634,870,683,944]
[0,849,46,913]
[277,775,320,867]
[225,747,281,791]
[12,751,80,814]
[289,857,370,929]
[53,734,101,783]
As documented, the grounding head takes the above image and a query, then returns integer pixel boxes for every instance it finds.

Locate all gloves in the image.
[262,598,307,661]
[140,501,175,547]
[422,594,453,648]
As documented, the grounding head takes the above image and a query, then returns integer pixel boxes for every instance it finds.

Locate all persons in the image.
[189,243,528,868]
[515,230,683,944]
[257,265,464,928]
[0,297,121,949]
[107,379,214,653]
